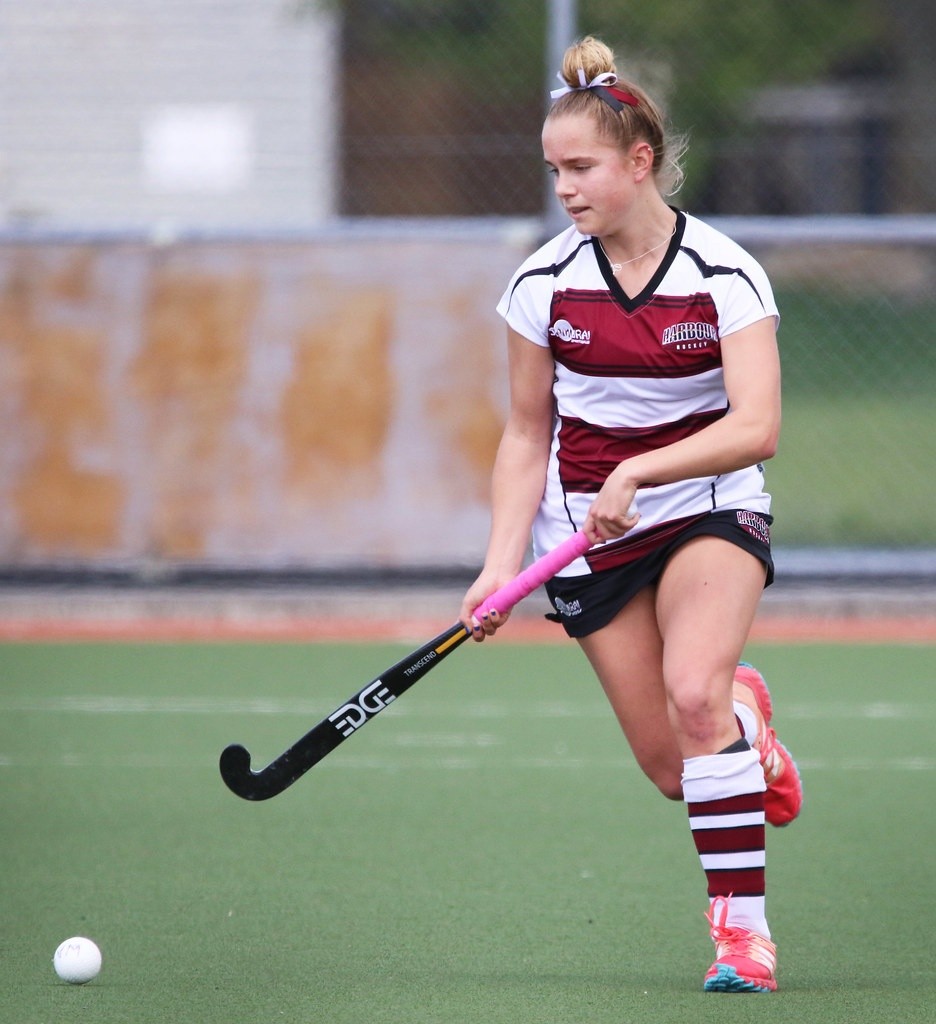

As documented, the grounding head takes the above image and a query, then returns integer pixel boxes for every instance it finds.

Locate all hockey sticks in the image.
[218,501,646,801]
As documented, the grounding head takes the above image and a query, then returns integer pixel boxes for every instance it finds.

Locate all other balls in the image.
[50,936,105,983]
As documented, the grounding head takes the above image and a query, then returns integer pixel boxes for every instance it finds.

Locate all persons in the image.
[457,35,802,993]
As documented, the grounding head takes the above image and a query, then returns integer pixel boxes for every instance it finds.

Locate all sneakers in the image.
[704,891,777,993]
[726,664,802,828]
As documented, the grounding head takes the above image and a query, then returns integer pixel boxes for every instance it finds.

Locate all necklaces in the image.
[598,225,677,270]
